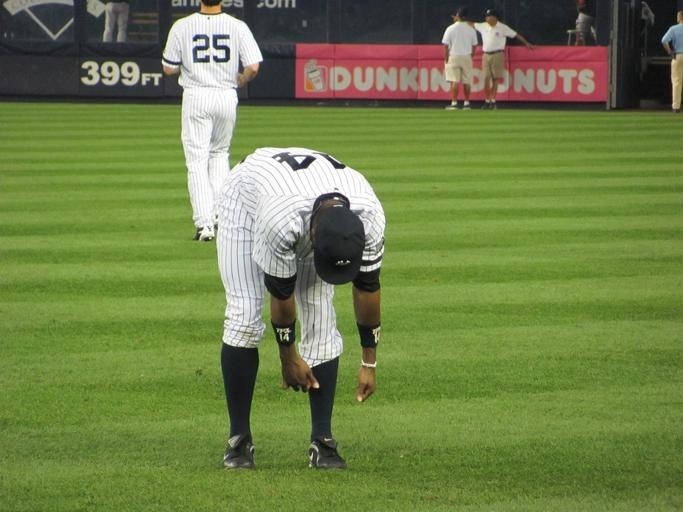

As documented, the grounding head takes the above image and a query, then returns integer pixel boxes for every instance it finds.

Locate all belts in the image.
[485,49,504,55]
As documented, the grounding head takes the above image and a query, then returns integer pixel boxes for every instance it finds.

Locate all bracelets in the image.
[355,320,381,347]
[268,320,296,349]
[358,360,377,369]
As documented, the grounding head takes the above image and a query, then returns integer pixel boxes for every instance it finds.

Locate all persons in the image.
[660,11,682,111]
[568,0,595,46]
[216,146,385,472]
[101,1,129,42]
[159,0,264,242]
[441,10,478,110]
[450,8,534,109]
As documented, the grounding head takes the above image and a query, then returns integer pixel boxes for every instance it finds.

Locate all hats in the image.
[314,207,365,284]
[483,9,499,17]
[456,6,469,19]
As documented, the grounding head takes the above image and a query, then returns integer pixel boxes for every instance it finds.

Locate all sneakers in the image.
[192,226,216,241]
[445,104,459,110]
[672,109,680,113]
[481,102,489,109]
[308,435,347,469]
[224,432,257,470]
[489,103,498,110]
[461,104,472,110]
